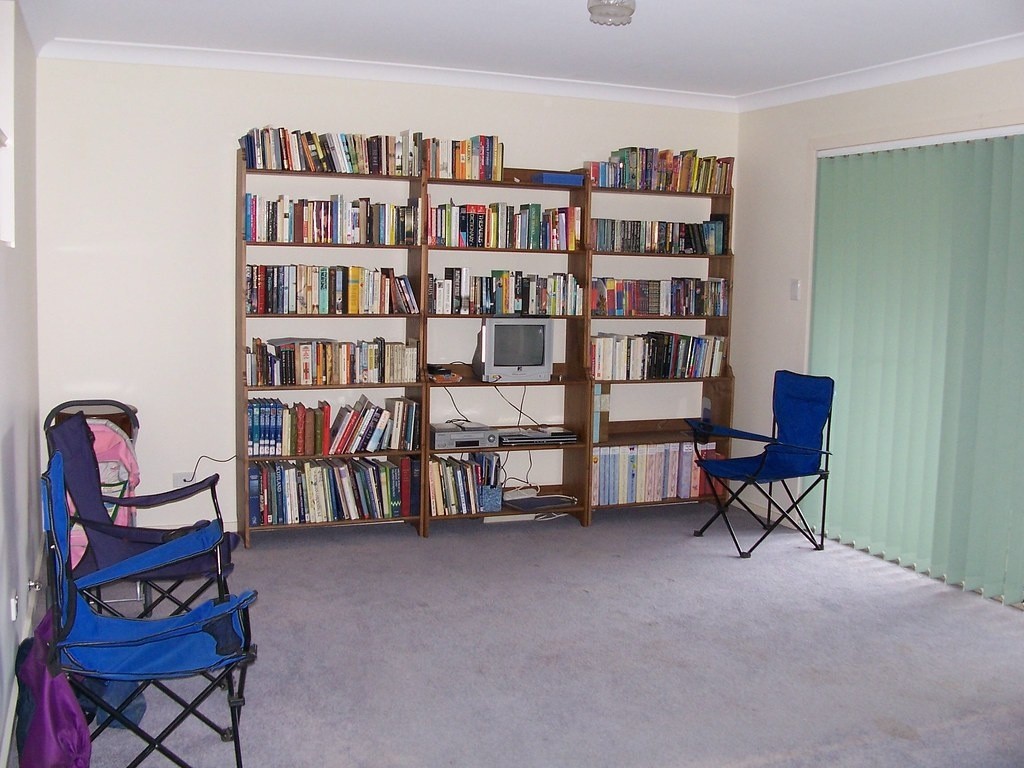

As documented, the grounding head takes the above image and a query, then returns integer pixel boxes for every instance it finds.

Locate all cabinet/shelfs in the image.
[236,147,735,550]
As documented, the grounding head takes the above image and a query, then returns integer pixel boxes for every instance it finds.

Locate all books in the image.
[427,267,584,315]
[590,332,726,381]
[592,384,610,443]
[245,193,422,245]
[246,337,419,386]
[246,265,419,315]
[584,145,735,195]
[590,213,728,255]
[428,453,502,516]
[246,397,421,457]
[239,125,505,182]
[591,277,729,316]
[430,371,463,383]
[248,459,420,527]
[591,441,725,507]
[427,195,581,251]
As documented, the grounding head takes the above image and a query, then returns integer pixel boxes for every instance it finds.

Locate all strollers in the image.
[43,399,153,618]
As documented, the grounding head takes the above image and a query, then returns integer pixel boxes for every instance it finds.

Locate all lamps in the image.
[586,1,635,26]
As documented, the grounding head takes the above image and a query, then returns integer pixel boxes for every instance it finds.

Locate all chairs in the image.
[15,411,260,767]
[683,370,836,557]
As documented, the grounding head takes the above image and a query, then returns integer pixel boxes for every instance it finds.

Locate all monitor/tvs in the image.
[471,318,553,383]
